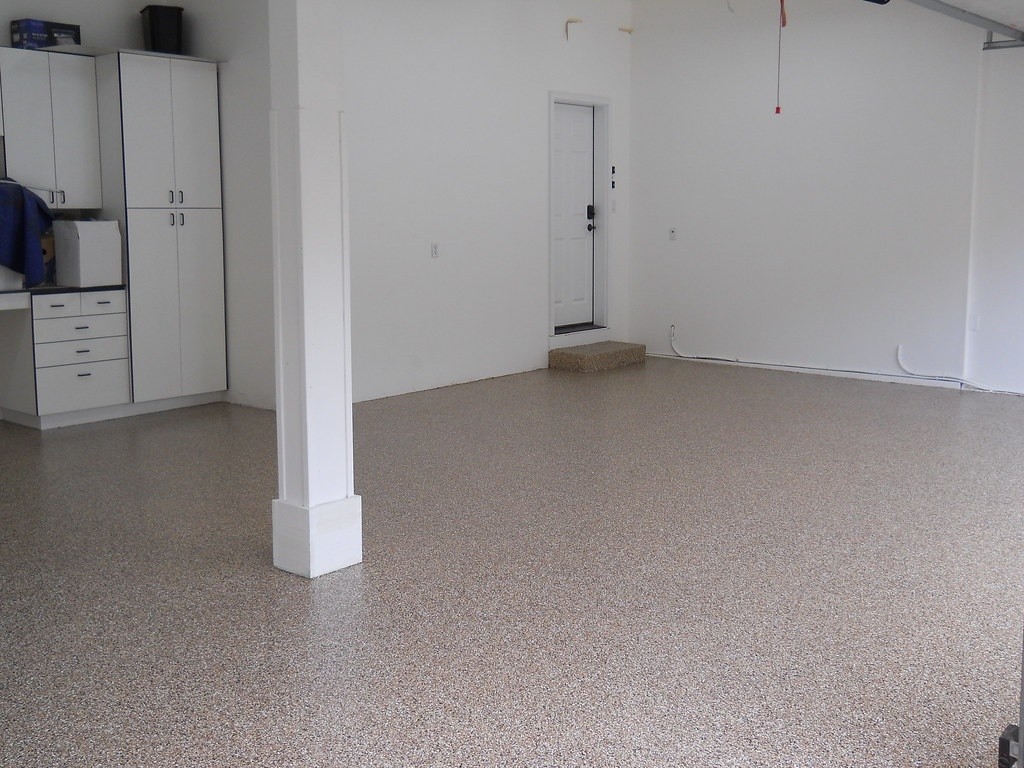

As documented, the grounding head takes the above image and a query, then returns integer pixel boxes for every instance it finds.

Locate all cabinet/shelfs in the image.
[0,290,133,430]
[96,47,228,416]
[0,45,103,211]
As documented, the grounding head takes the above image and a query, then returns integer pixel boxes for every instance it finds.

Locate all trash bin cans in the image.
[139,4,184,54]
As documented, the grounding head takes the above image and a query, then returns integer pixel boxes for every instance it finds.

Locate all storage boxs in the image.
[139,5,185,52]
[11,19,81,49]
